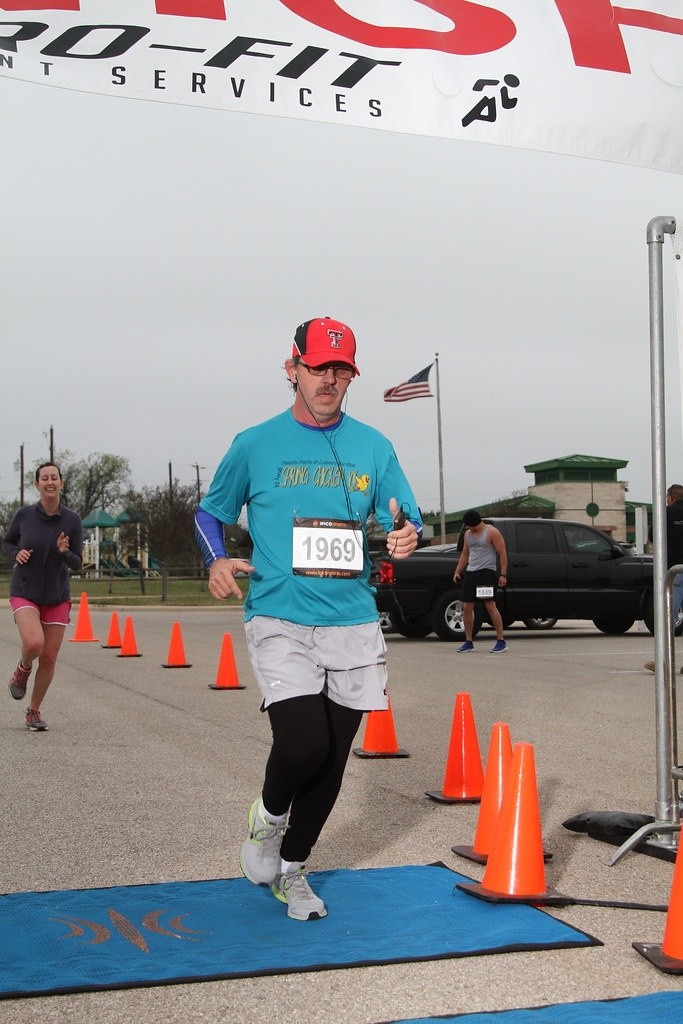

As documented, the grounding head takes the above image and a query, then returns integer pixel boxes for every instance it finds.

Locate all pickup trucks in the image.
[369,518,654,642]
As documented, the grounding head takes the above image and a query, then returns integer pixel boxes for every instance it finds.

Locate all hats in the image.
[293,317,361,377]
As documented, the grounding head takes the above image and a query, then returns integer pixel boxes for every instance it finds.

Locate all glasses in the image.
[298,362,354,380]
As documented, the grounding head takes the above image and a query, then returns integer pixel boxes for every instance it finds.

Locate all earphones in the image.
[293,371,298,381]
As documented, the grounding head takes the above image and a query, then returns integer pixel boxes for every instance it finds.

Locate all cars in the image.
[378,544,458,634]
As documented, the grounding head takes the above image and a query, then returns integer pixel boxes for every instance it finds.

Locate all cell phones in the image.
[394,511,405,532]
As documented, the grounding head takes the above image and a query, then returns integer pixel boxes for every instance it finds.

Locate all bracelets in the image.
[501,575,506,577]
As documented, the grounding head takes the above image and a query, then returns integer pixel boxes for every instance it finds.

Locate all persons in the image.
[645,484,683,673]
[195,316,425,921]
[455,512,508,653]
[4,464,84,731]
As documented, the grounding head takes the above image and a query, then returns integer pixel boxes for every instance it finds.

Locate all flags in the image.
[384,362,434,402]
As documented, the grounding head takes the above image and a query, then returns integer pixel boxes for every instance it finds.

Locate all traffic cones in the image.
[161,622,192,668]
[101,613,122,648]
[68,592,99,642]
[352,689,411,760]
[425,692,484,804]
[208,633,247,690]
[455,741,575,906]
[117,616,143,657]
[631,819,683,975]
[451,720,553,866]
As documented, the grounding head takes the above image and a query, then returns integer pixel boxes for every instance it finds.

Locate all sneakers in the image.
[456,639,475,653]
[271,860,328,921]
[239,797,291,885]
[25,708,48,731]
[490,639,508,652]
[7,655,32,700]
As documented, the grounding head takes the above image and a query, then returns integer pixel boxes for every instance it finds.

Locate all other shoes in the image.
[645,660,655,673]
